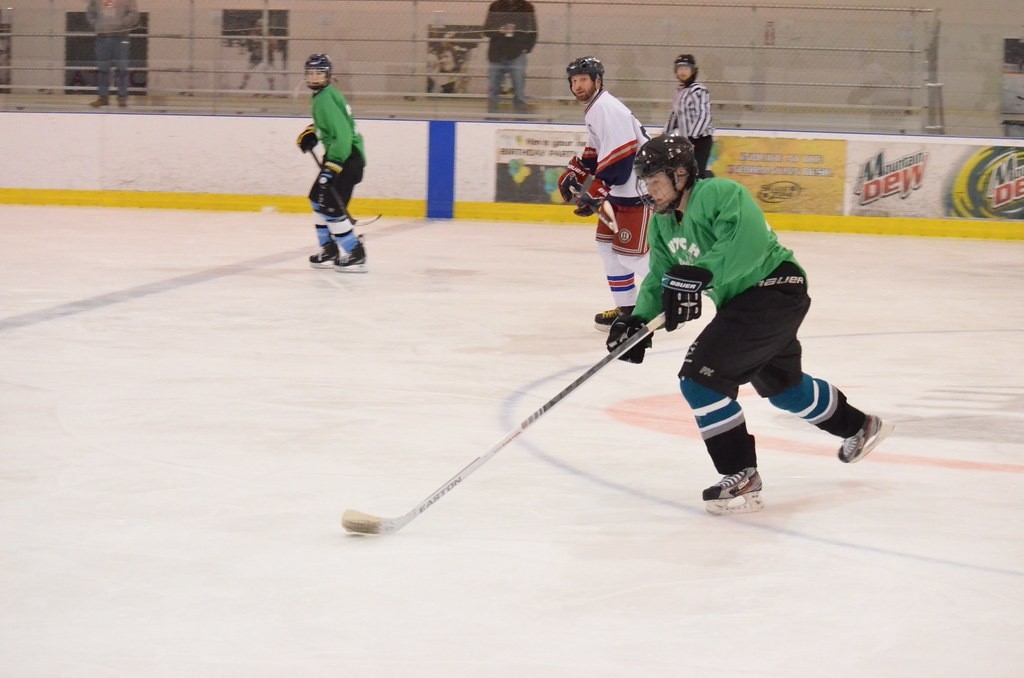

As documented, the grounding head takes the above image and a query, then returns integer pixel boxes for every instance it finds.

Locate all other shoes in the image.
[117,97,128,106]
[91,99,109,106]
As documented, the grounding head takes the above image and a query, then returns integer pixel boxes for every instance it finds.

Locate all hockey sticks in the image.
[566,184,620,237]
[341,310,669,534]
[308,148,384,227]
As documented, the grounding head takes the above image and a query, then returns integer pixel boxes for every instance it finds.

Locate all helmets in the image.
[567,56,604,75]
[304,54,333,90]
[634,137,698,214]
[674,54,696,67]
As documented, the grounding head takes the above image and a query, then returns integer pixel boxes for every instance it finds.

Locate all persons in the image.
[557,57,653,333]
[297,52,369,272]
[483,0,539,119]
[665,54,714,181]
[240,18,278,97]
[86,0,140,107]
[604,135,895,515]
[428,28,513,96]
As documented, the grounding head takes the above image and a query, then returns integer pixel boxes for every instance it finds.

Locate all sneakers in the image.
[310,244,337,263]
[335,241,365,266]
[839,415,882,463]
[595,306,636,325]
[703,467,762,501]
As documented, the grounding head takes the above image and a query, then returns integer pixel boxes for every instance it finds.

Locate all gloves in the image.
[558,156,589,203]
[606,314,653,364]
[316,160,343,191]
[298,124,318,153]
[661,264,713,332]
[574,178,611,216]
